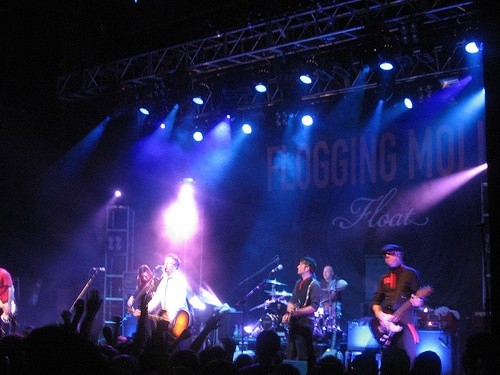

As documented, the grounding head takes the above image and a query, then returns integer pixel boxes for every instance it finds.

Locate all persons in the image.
[281,257,320,361]
[320,266,348,318]
[371,245,423,372]
[0,268,16,334]
[127,255,191,332]
[0,289,500,375]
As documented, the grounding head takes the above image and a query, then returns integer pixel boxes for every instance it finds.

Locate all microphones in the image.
[158,265,167,268]
[276,297,284,303]
[93,266,105,271]
[271,264,283,272]
[247,282,266,296]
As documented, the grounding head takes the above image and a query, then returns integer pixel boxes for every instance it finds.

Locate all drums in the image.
[262,315,284,331]
[313,300,342,341]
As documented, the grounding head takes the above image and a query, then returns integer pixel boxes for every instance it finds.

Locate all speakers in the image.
[363,254,391,317]
[415,330,462,375]
[347,321,381,353]
[121,271,137,339]
[218,312,244,342]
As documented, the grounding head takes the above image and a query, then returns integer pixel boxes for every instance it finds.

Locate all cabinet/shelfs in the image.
[103,205,133,342]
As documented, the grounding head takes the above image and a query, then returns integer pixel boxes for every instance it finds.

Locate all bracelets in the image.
[285,312,290,314]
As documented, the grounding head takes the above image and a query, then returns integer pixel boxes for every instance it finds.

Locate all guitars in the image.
[282,303,300,328]
[0,300,16,324]
[124,307,189,337]
[369,287,433,350]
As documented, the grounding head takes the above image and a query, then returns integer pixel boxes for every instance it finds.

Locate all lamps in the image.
[115,189,125,199]
[255,66,271,93]
[193,124,208,142]
[404,92,420,110]
[191,79,212,105]
[242,119,256,134]
[299,55,318,85]
[376,74,395,104]
[300,108,315,126]
[139,96,153,116]
[377,42,396,71]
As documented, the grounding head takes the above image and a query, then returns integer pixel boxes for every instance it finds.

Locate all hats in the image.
[381,245,401,252]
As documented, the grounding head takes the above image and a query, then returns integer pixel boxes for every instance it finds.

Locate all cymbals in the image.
[322,288,332,292]
[267,280,287,287]
[265,291,293,296]
